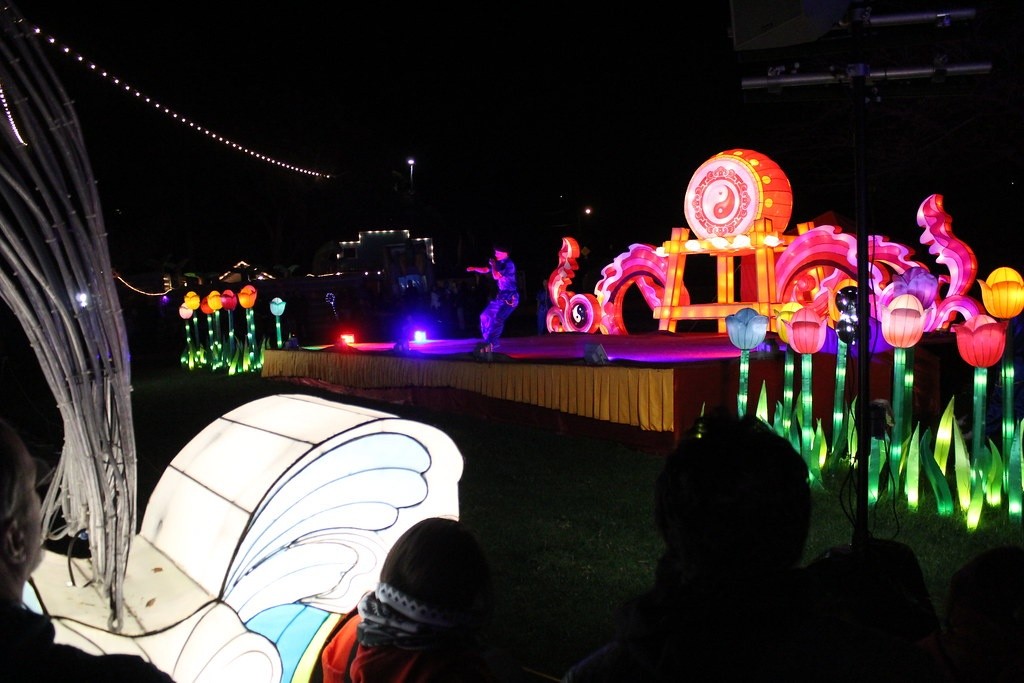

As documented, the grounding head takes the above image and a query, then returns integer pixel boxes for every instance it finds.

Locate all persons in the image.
[397,252,439,293]
[467,245,519,353]
[0,420,176,683]
[321,518,488,683]
[560,415,1024,683]
[536,278,553,336]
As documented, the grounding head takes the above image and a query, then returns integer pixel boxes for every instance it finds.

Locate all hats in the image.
[374,518,495,631]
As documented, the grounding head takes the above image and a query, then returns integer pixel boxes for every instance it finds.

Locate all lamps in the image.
[583,342,609,365]
[473,342,493,362]
[394,339,409,353]
[285,338,298,349]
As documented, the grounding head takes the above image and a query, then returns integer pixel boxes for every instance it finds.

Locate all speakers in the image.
[730,0,850,52]
